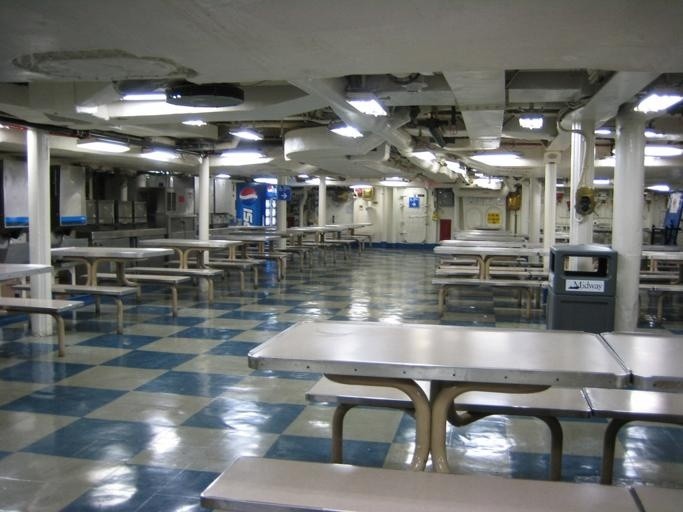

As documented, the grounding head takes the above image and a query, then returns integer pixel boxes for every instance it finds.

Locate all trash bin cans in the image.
[546,244,617,334]
